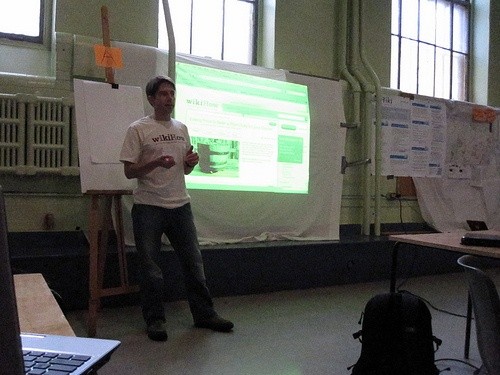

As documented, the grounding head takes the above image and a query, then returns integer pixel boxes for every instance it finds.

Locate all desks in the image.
[390,233,500,359]
[12,273,77,337]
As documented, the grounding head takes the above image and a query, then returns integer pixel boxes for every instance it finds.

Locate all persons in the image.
[119,75,235,342]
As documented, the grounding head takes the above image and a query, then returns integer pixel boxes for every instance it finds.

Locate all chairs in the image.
[457,256,500,375]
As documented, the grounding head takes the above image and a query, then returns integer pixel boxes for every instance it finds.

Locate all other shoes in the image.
[146,321,167,341]
[193,312,234,331]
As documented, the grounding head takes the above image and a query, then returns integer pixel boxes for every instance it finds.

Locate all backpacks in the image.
[351,292,442,375]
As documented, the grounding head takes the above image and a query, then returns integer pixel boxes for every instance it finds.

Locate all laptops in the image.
[0,186,121,375]
